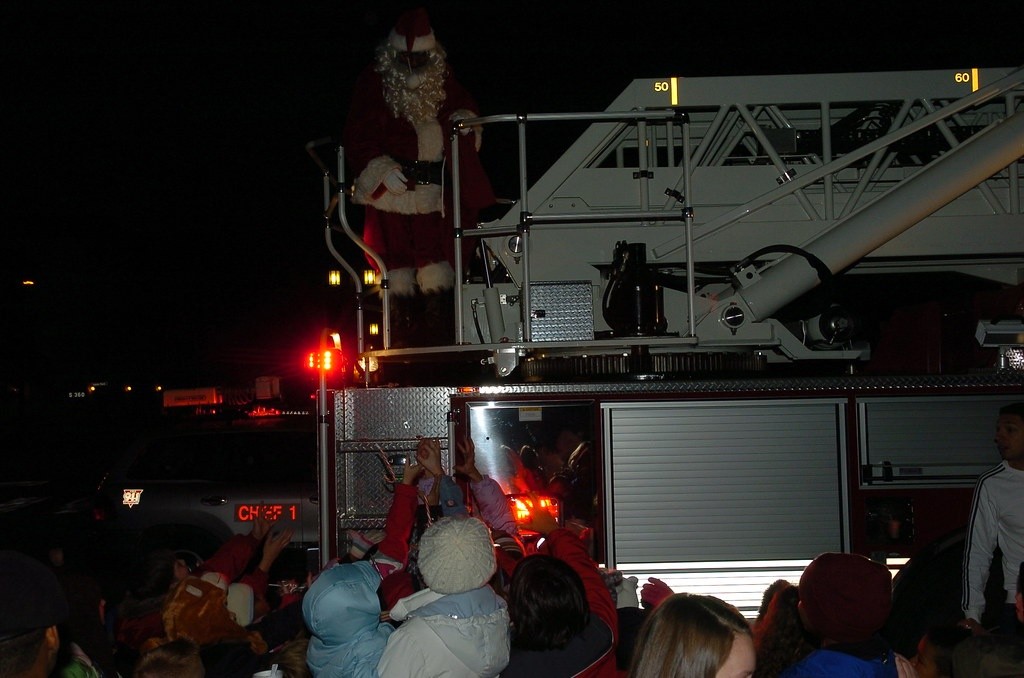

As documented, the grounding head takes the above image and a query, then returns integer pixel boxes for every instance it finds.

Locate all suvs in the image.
[95,409,323,594]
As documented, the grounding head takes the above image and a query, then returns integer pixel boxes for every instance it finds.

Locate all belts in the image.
[389,156,443,186]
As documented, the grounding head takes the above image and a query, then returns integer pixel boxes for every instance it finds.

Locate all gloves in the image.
[454,116,471,135]
[641,578,674,606]
[382,167,409,196]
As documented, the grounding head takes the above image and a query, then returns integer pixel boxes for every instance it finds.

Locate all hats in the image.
[162,576,268,655]
[388,6,435,89]
[0,548,70,641]
[416,515,497,594]
[800,553,893,645]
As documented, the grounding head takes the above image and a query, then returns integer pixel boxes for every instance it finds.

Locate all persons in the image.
[0,403,1024,678]
[346,4,493,388]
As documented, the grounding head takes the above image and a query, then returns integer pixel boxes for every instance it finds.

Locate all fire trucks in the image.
[320,67,1023,665]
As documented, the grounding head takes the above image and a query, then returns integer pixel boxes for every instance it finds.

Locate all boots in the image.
[379,261,454,348]
